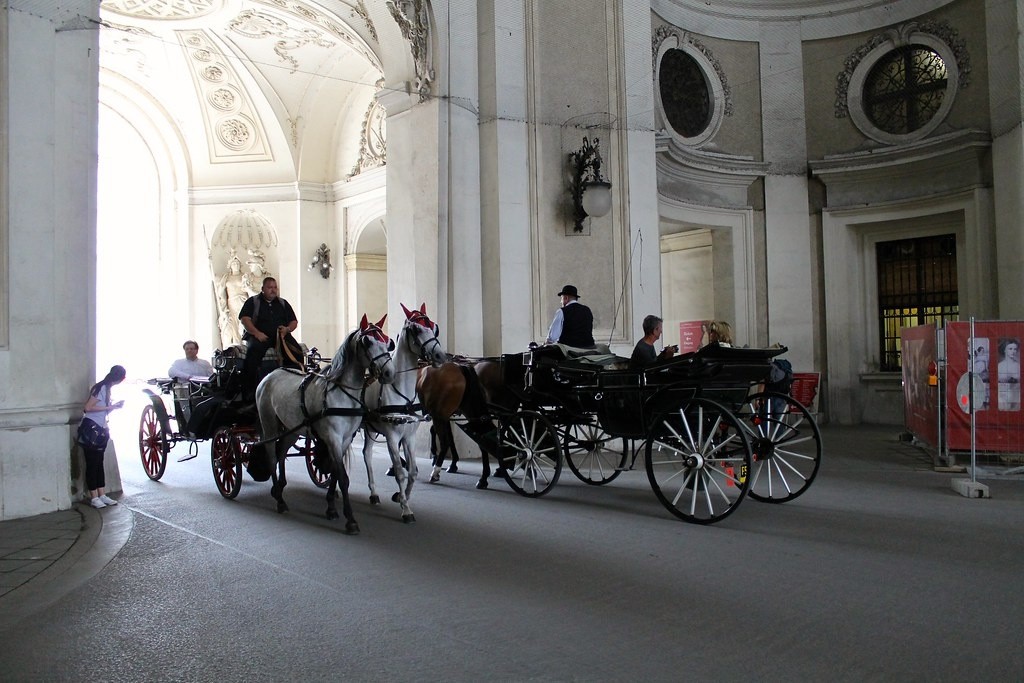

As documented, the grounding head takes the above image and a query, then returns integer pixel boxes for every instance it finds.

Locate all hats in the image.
[558,285,581,298]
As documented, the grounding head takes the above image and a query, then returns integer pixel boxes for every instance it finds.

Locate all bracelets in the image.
[286,327,291,331]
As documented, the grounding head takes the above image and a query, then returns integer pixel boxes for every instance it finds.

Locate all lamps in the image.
[308,242,335,280]
[569,135,614,233]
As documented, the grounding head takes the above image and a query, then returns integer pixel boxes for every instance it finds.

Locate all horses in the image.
[342,302,448,524]
[385,354,537,489]
[255,313,397,536]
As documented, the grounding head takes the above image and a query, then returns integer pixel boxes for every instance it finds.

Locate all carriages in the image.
[138,302,446,535]
[412,340,823,525]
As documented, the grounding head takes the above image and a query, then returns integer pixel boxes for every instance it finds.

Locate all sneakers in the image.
[90,498,107,510]
[100,495,118,505]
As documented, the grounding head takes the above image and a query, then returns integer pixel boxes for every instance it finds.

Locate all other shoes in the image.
[238,401,257,412]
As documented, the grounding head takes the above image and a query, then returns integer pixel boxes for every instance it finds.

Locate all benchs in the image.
[528,339,788,436]
[148,342,310,441]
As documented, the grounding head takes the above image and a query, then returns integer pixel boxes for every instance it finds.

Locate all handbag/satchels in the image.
[71,416,110,447]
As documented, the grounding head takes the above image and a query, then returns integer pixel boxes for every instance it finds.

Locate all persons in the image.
[999,339,1020,383]
[700,324,709,349]
[168,339,214,393]
[967,343,989,381]
[238,276,304,399]
[626,315,679,386]
[242,252,272,298]
[542,286,597,350]
[74,364,126,510]
[705,318,733,346]
[755,358,785,443]
[215,254,249,349]
